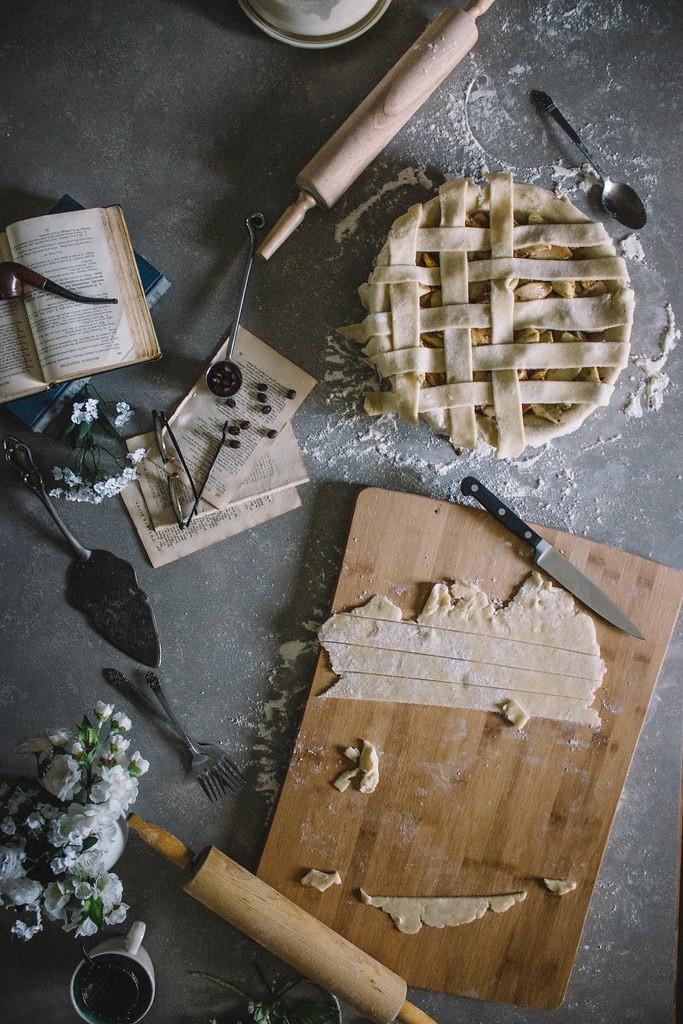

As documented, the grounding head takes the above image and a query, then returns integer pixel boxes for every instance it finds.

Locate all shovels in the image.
[1,434,164,671]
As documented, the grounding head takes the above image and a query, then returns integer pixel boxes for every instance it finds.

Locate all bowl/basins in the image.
[237,0,393,50]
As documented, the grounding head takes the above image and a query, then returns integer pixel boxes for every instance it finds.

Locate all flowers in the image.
[0,701,149,939]
[48,383,147,507]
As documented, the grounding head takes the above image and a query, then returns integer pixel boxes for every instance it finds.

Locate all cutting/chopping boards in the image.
[255,487,683,1009]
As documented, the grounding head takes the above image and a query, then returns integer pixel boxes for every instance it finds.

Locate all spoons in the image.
[526,88,647,230]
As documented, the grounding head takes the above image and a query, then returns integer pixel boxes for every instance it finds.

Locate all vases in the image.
[91,808,129,872]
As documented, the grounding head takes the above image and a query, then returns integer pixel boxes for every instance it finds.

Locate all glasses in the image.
[152,409,229,529]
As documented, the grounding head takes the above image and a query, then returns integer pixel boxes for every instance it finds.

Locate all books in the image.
[0,204,162,406]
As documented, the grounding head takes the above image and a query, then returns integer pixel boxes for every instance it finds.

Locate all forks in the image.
[145,670,227,804]
[101,667,248,795]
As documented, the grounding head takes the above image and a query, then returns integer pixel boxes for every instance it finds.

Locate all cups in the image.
[70,921,156,1024]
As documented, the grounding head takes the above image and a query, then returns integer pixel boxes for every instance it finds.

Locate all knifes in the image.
[460,475,648,643]
